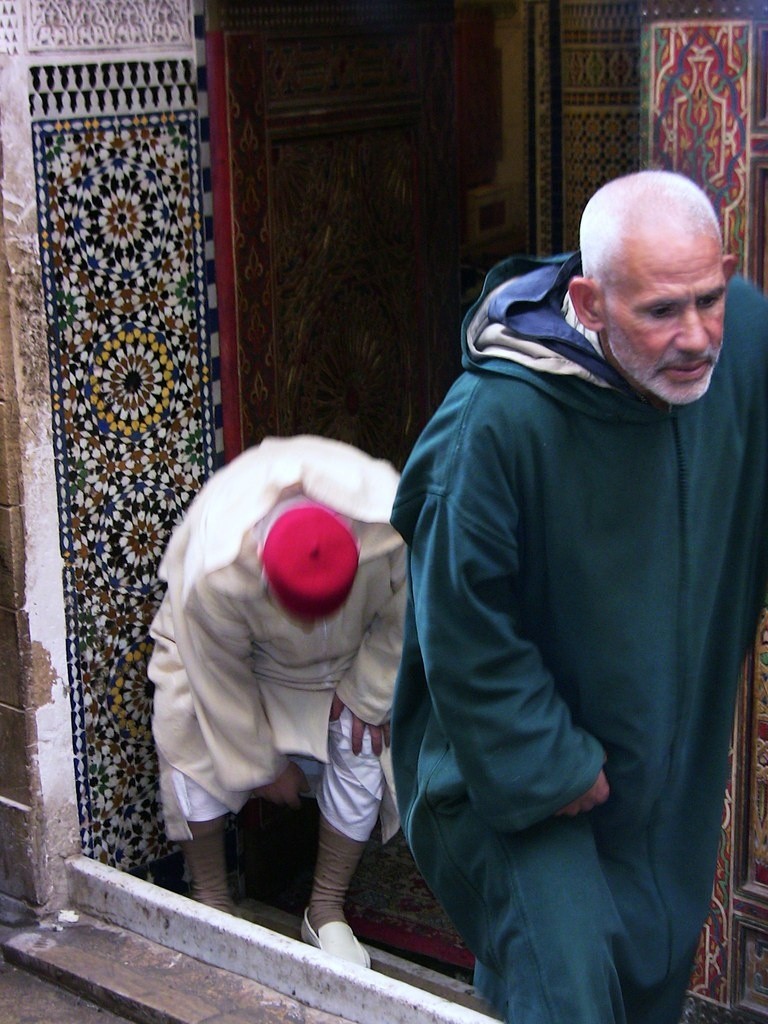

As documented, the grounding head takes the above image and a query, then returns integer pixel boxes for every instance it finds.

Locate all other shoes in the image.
[300,906,370,970]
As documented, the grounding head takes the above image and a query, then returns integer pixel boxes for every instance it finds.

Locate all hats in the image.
[261,505,359,621]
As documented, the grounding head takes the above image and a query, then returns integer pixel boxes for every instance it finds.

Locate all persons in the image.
[389,169,768,1024]
[147,434,411,972]
